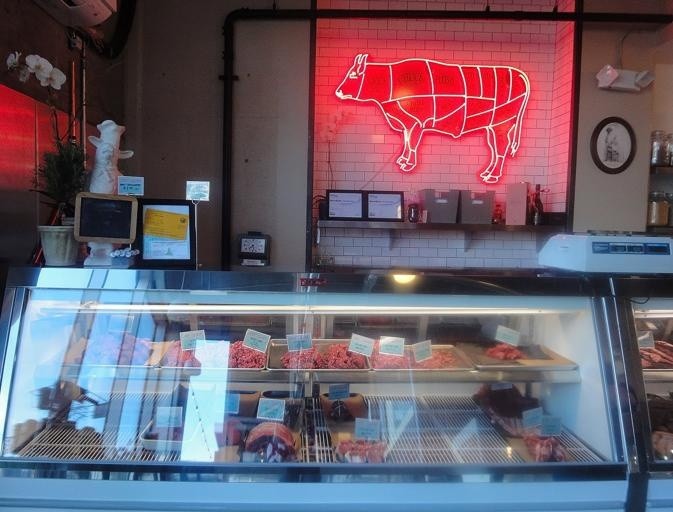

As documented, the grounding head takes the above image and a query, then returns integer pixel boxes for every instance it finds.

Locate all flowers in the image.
[5,50,86,224]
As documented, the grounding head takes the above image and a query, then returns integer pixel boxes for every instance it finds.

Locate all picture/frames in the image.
[325,190,405,221]
[133,198,195,270]
[590,116,638,175]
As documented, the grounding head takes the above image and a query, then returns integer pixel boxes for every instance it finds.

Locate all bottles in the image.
[648,189,673,227]
[651,128,673,166]
[532,184,543,225]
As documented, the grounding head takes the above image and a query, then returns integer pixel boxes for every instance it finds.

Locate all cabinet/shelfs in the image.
[1,270,672,511]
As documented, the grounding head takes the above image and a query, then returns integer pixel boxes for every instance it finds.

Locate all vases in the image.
[38,225,80,266]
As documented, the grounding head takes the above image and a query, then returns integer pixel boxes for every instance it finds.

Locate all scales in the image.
[537,229,673,273]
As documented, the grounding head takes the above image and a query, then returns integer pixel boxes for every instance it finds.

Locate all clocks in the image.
[238,233,271,267]
